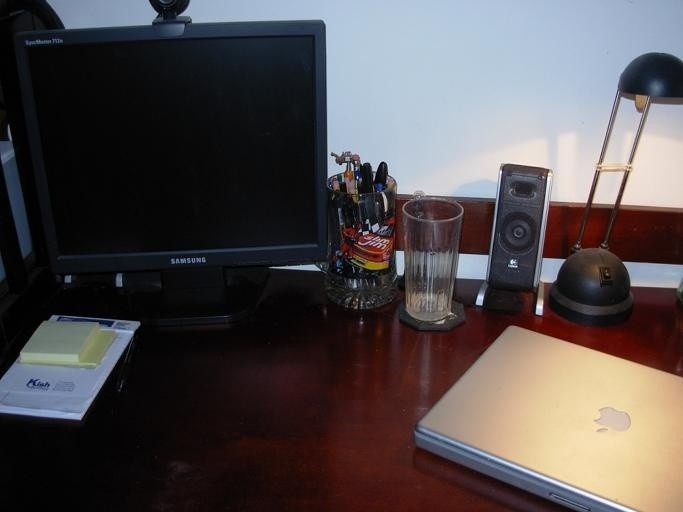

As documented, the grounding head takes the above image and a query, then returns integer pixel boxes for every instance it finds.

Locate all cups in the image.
[327,170,396,310]
[401,197,465,323]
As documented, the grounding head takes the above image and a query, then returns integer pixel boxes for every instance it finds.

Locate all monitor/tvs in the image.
[14,19,333,329]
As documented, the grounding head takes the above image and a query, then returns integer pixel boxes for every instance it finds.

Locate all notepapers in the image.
[21,321,118,367]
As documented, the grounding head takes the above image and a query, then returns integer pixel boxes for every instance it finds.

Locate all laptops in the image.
[413,322,682,512]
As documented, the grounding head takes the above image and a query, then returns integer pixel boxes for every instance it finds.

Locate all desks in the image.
[1,268,682,512]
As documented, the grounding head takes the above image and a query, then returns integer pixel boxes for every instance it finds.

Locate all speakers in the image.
[474,161,555,320]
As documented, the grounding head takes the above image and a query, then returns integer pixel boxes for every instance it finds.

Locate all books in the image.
[18,329,118,367]
[18,319,98,365]
[0,314,139,420]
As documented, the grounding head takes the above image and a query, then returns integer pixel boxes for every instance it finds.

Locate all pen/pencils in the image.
[333,162,389,279]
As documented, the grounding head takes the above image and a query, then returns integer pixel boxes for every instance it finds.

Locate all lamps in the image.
[548,52,683,329]
